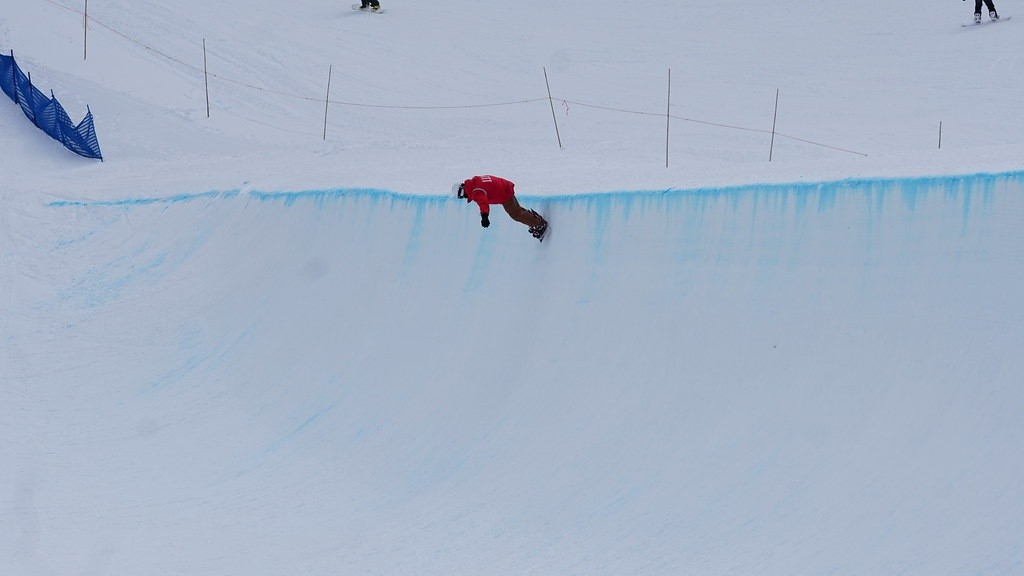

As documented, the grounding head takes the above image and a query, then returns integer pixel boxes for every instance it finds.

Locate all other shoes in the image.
[360,6,366,10]
[371,7,382,13]
[989,11,999,20]
[974,13,981,22]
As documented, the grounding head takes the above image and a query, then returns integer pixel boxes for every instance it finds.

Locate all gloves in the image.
[480,212,490,228]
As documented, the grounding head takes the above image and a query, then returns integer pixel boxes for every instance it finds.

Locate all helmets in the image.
[451,181,463,199]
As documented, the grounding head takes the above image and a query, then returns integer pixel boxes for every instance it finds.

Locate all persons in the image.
[451,174,552,242]
[973,0,1000,23]
[360,0,380,12]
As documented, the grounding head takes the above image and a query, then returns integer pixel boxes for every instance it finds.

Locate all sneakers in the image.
[529,217,548,238]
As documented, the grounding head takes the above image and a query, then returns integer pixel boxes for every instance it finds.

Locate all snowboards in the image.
[526,207,554,246]
